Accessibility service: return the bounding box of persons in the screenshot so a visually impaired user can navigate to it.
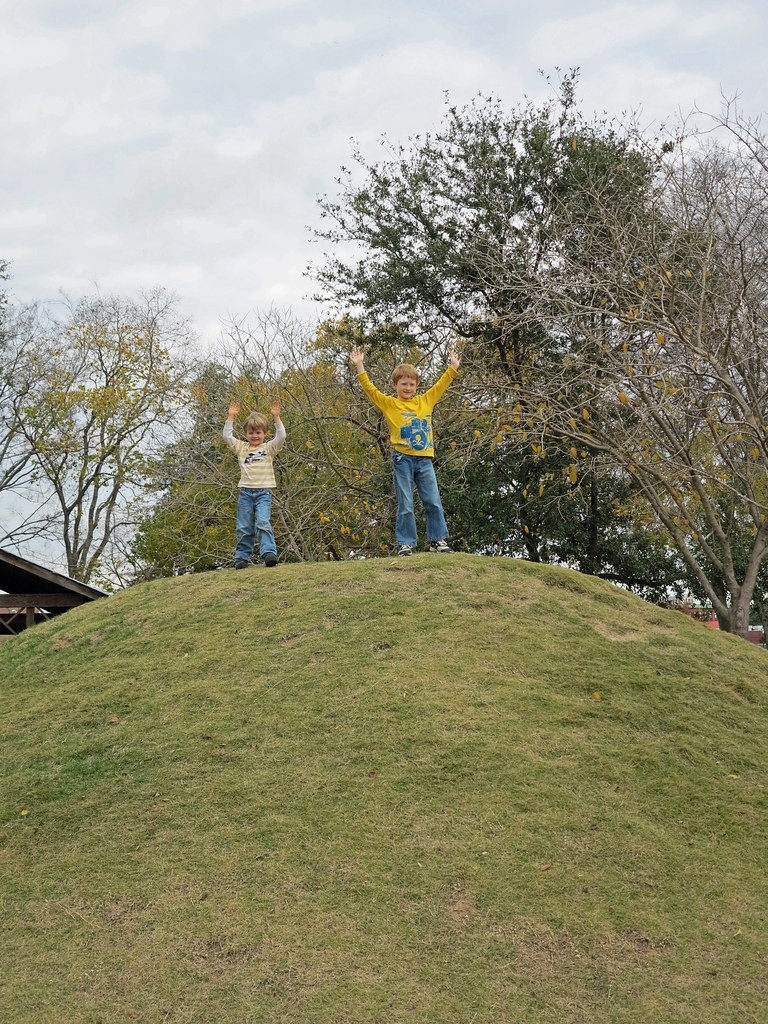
[350,347,461,555]
[223,401,286,570]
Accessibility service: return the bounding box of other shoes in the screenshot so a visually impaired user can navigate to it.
[235,558,248,570]
[265,552,278,567]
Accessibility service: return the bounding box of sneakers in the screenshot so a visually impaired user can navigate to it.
[398,544,413,556]
[429,539,452,552]
